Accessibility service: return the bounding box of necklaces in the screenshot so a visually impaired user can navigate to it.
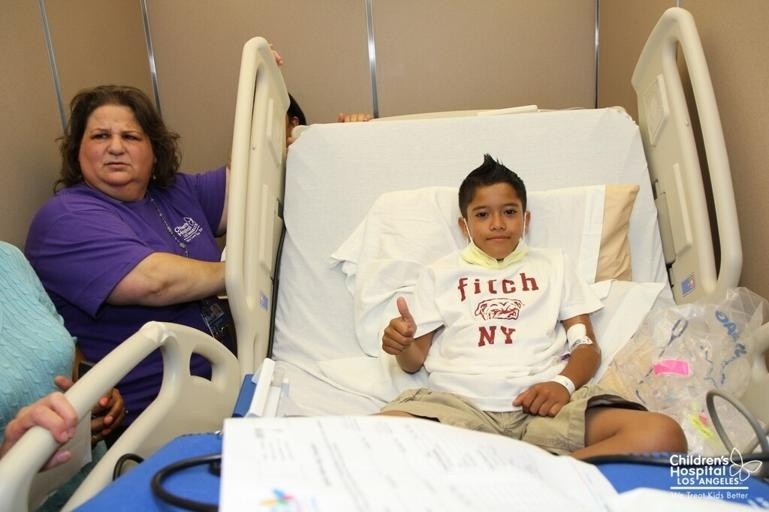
[147,192,190,258]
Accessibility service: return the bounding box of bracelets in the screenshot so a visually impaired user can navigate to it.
[553,375,576,395]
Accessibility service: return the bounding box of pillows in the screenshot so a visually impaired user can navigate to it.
[330,184,644,361]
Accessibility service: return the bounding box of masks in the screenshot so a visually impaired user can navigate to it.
[459,210,530,271]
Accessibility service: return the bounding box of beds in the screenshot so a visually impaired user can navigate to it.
[0,4,769,507]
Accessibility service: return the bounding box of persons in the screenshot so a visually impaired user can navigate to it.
[284,92,375,149]
[382,152,689,458]
[0,239,127,510]
[23,43,283,450]
[0,392,76,471]
